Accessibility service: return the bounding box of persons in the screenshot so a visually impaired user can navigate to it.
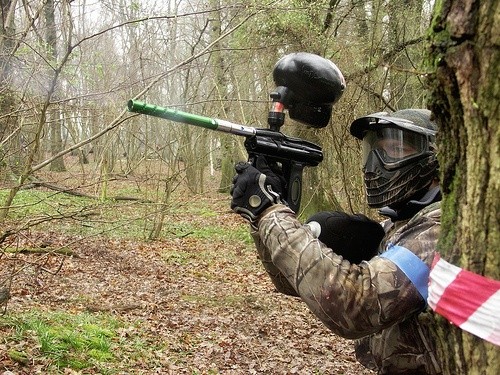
[229,107,445,375]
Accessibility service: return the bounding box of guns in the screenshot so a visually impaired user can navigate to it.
[126,52,346,216]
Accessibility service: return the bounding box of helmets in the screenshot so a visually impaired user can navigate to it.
[350,108,441,209]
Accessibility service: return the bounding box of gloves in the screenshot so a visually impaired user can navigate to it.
[229,154,288,231]
